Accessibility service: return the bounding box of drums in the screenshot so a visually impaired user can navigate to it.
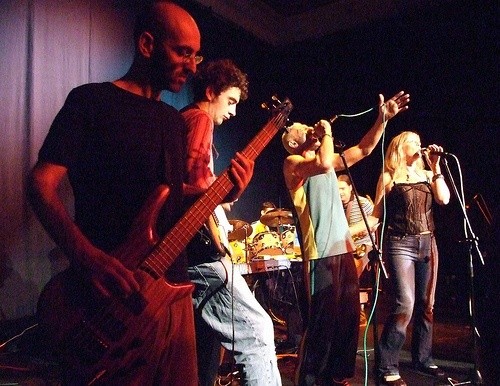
[281,228,300,255]
[249,230,284,258]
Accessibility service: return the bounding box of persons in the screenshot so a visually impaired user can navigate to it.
[372,131,451,386]
[281,90,410,386]
[176,57,283,386]
[24,2,252,386]
[336,175,378,281]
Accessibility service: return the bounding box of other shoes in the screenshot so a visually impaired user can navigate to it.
[329,376,353,386]
[276,342,300,354]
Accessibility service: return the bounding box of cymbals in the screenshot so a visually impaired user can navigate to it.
[226,219,254,242]
[261,211,294,225]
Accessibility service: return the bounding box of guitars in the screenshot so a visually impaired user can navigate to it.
[36,93,294,381]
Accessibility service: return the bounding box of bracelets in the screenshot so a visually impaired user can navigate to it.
[322,134,334,141]
[432,174,444,183]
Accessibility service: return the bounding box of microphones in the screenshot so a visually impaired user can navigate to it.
[466,193,479,208]
[312,115,338,138]
[420,148,453,156]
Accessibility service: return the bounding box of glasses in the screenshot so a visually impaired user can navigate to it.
[154,37,203,65]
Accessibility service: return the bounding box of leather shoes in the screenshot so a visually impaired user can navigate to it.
[381,375,407,386]
[419,365,446,376]
